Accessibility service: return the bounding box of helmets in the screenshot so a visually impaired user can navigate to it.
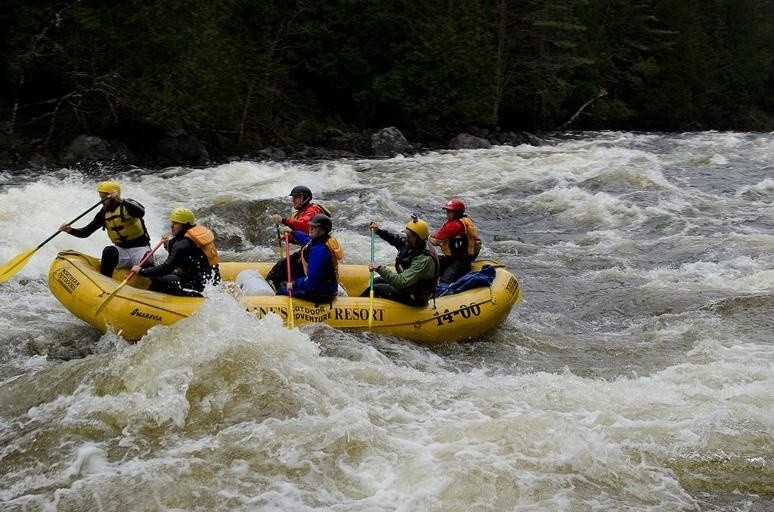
[307,214,332,232]
[97,181,121,194]
[289,186,312,199]
[442,199,465,212]
[169,209,195,225]
[406,219,429,240]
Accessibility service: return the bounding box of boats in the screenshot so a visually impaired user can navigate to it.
[49,250,520,344]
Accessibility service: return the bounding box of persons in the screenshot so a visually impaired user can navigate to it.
[429,199,480,285]
[359,219,439,307]
[276,214,343,304]
[265,185,332,290]
[58,182,155,277]
[130,208,221,297]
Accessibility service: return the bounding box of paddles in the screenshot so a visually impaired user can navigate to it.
[284,232,294,328]
[0,190,118,283]
[94,235,169,315]
[369,221,373,329]
